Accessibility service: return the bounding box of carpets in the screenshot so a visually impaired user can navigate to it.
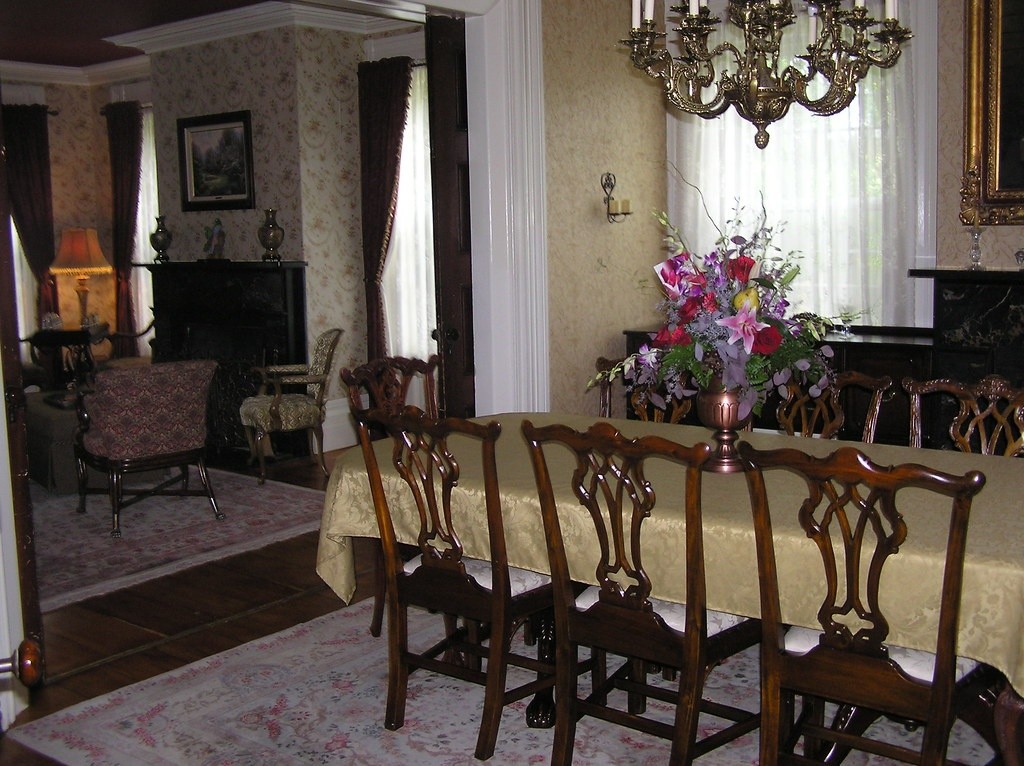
[5,588,997,766]
[29,463,326,612]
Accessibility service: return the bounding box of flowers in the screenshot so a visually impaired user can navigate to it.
[582,185,860,383]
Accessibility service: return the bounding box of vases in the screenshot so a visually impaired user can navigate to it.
[258,208,285,261]
[150,217,170,261]
[966,228,986,265]
[695,354,752,472]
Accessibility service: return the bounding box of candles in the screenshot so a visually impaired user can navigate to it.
[974,210,979,228]
[608,197,630,213]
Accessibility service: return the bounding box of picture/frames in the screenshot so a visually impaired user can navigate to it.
[177,109,255,212]
[959,0,1024,225]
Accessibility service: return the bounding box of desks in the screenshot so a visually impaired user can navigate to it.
[31,323,107,395]
[315,414,1024,691]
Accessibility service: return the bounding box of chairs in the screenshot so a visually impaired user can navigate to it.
[241,327,1024,765]
[69,361,225,536]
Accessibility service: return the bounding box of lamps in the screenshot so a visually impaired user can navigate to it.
[51,227,112,326]
[617,0,914,149]
